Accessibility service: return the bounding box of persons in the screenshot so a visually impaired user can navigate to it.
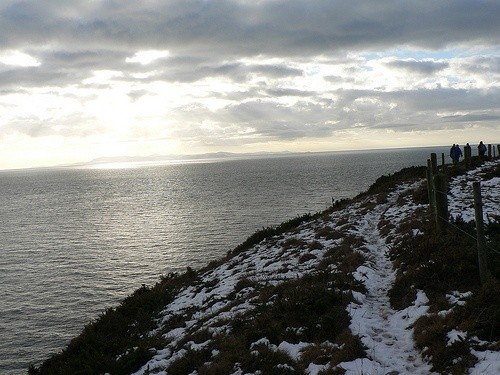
[466,142,471,157]
[478,141,487,157]
[450,144,457,164]
[455,145,463,163]
